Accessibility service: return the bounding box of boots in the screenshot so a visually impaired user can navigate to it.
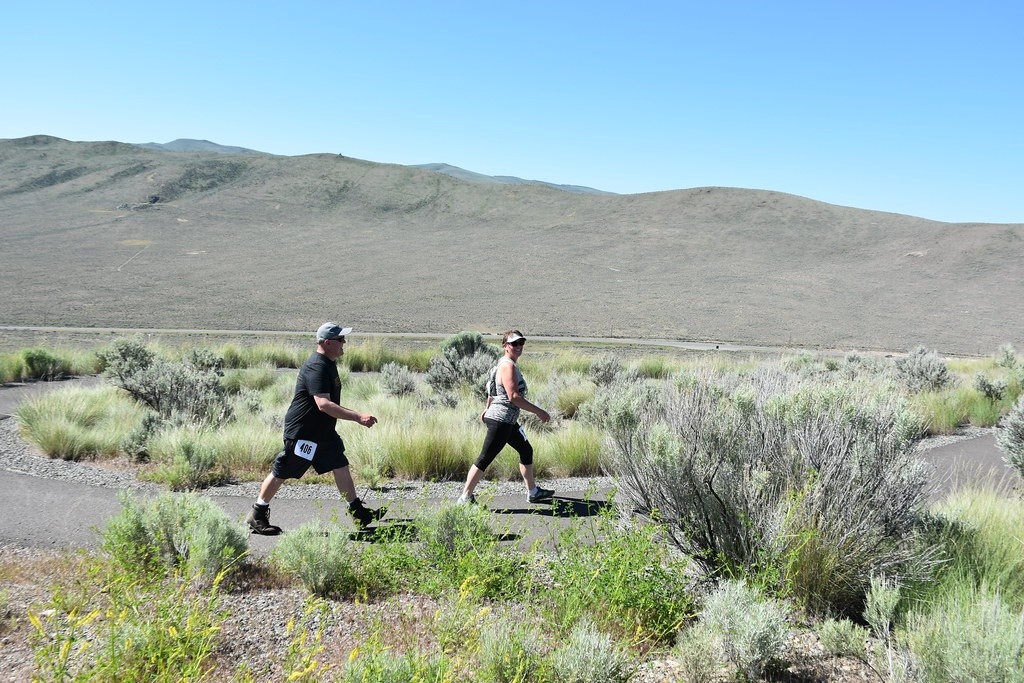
[347,498,389,530]
[242,503,284,535]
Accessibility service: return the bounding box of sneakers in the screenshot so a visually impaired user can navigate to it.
[457,494,487,510]
[526,487,555,503]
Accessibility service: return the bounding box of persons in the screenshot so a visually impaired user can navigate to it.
[246,323,387,535]
[458,330,555,507]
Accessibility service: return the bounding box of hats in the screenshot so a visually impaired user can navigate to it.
[316,322,352,343]
[505,332,526,342]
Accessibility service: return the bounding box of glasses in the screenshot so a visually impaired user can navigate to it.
[323,335,344,344]
[506,340,525,347]
